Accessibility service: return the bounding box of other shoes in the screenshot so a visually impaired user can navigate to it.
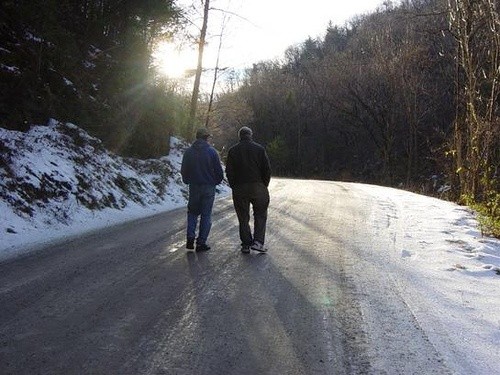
[251,241,268,253]
[195,244,210,253]
[240,245,250,254]
[185,241,194,249]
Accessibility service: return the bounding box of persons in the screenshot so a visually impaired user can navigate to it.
[181,130,224,253]
[225,126,271,254]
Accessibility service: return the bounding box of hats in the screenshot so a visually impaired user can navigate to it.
[238,127,254,138]
[195,127,213,138]
